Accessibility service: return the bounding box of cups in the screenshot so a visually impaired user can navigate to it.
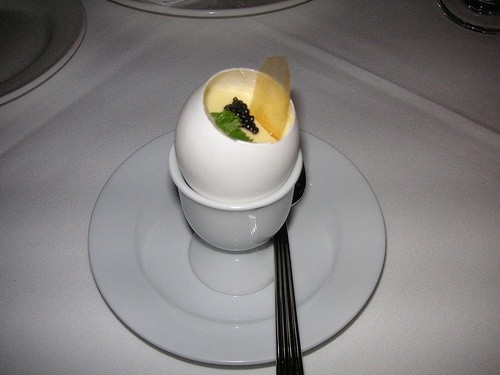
[169,142,303,297]
[436,0,500,37]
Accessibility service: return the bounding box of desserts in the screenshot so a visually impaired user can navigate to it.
[206,81,292,143]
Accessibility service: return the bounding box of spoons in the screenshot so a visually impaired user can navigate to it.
[274,162,306,375]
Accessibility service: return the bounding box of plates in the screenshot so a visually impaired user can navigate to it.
[109,0,311,17]
[0,0,89,103]
[88,129,387,365]
